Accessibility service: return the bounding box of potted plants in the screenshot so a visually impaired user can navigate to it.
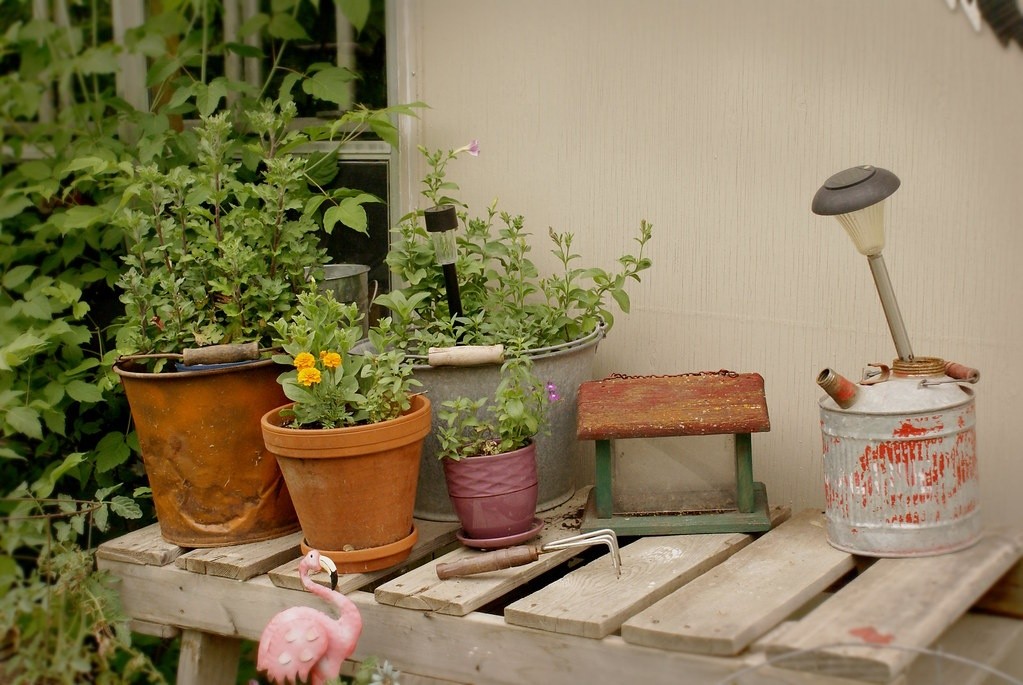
[257,274,435,577]
[110,96,336,551]
[434,335,562,550]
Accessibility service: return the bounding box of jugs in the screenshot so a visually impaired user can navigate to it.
[815,356,986,557]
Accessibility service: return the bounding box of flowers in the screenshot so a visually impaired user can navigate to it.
[284,347,369,431]
[346,136,658,360]
[523,378,562,444]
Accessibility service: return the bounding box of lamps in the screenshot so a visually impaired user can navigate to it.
[422,204,460,266]
[812,164,902,258]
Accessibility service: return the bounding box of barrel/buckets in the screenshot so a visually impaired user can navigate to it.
[301,264,372,339]
